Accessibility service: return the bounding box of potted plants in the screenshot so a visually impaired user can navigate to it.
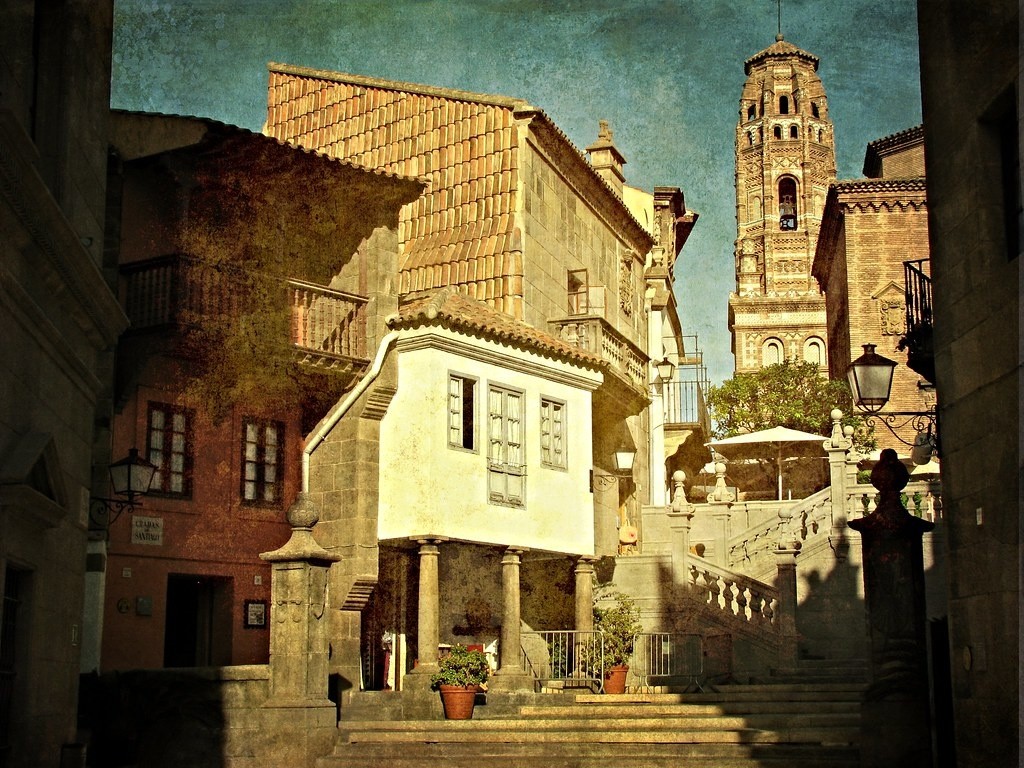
[580,593,645,695]
[429,643,490,720]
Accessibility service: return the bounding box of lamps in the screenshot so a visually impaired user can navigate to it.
[843,342,943,460]
[593,440,637,491]
[88,446,160,528]
[649,357,676,385]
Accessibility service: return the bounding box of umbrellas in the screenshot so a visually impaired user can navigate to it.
[849,447,912,467]
[909,459,940,481]
[703,425,828,501]
